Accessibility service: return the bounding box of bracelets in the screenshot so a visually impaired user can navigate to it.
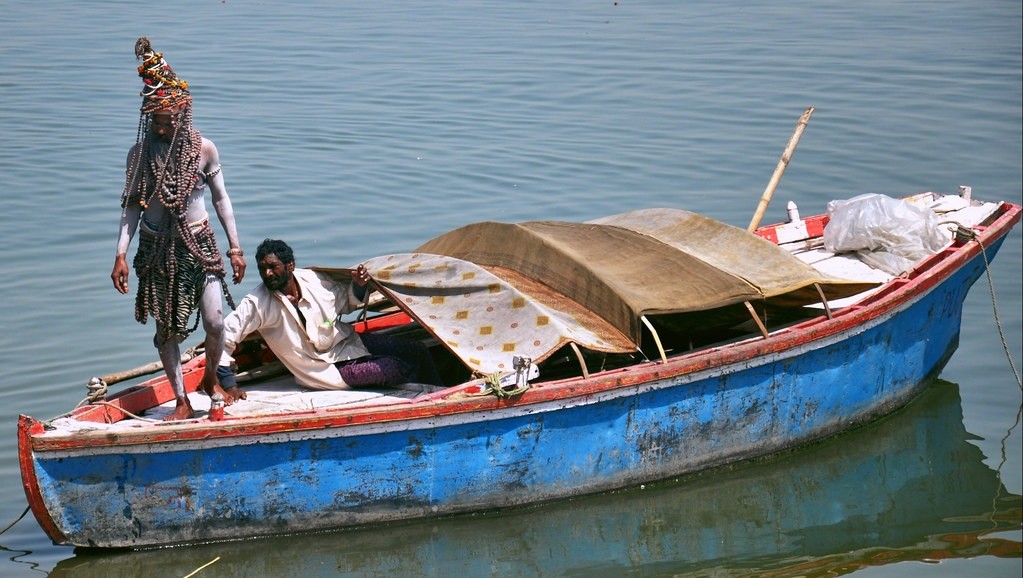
[226,248,244,258]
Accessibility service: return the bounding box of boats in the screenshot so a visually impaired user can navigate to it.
[15,189,1023,550]
[30,376,1021,577]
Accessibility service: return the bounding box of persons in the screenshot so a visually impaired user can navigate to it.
[216,238,446,403]
[111,90,247,422]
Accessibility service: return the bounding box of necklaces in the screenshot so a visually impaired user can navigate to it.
[141,129,203,220]
